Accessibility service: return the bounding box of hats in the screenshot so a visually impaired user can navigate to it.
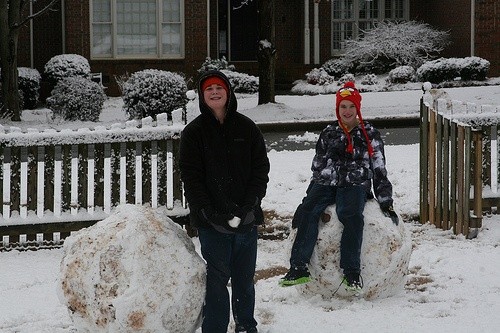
[334,81,361,117]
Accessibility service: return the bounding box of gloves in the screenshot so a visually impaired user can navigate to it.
[381,200,399,225]
[291,202,305,229]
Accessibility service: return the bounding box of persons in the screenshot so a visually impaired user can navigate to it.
[282,81,399,291]
[178,70,270,333]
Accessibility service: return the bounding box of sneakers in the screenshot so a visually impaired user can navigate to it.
[278,266,311,285]
[342,273,361,295]
